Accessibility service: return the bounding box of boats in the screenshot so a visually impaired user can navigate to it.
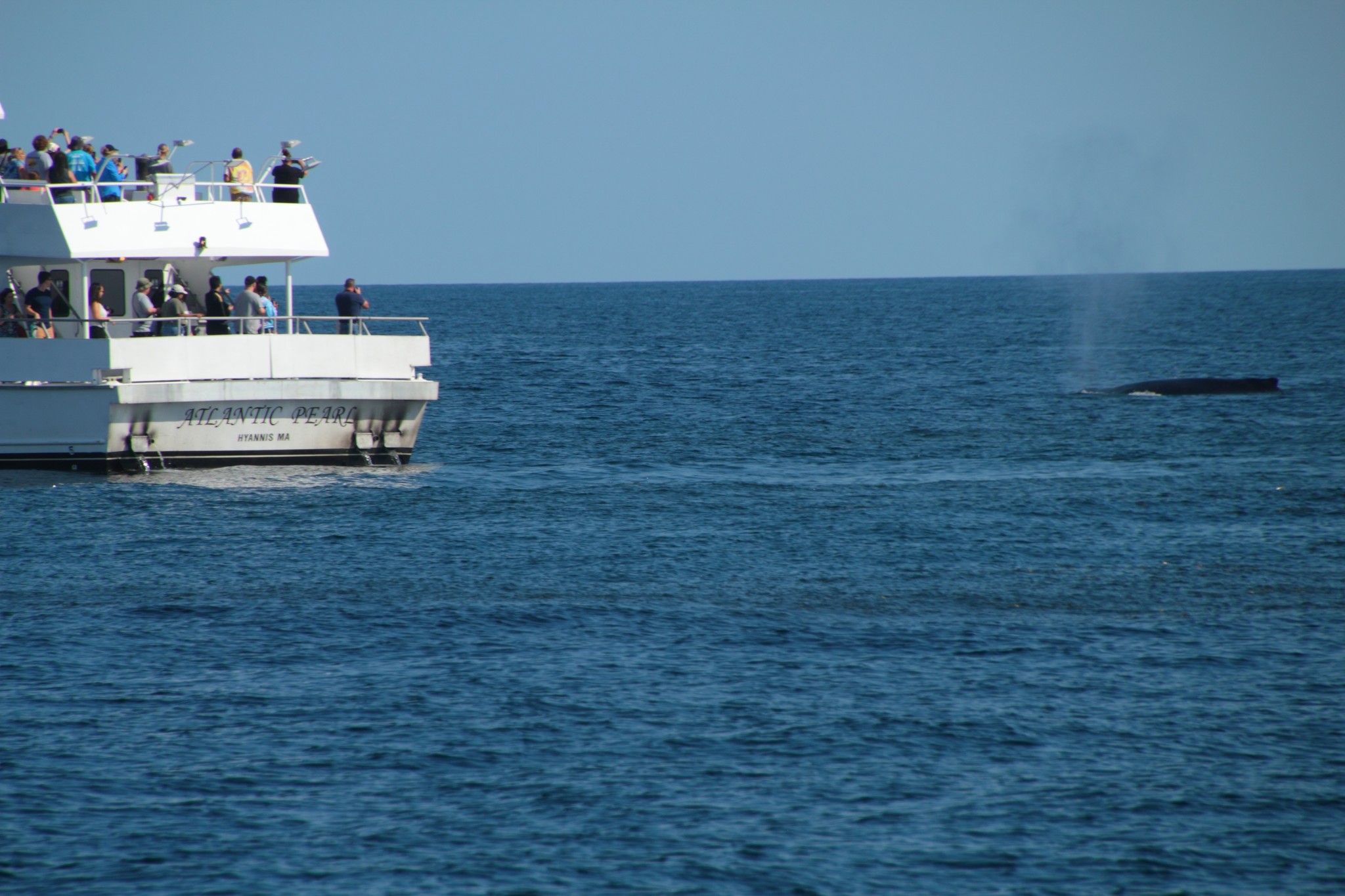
[0,136,444,479]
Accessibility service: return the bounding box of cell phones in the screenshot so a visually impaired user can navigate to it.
[57,128,63,134]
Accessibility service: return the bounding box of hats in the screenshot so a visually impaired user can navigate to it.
[67,135,86,150]
[171,284,189,294]
[245,276,258,285]
[47,142,60,153]
[135,277,154,290]
[102,144,119,154]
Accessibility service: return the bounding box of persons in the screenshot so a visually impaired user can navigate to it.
[223,147,254,203]
[271,152,309,203]
[0,269,279,338]
[335,278,369,335]
[0,127,174,204]
